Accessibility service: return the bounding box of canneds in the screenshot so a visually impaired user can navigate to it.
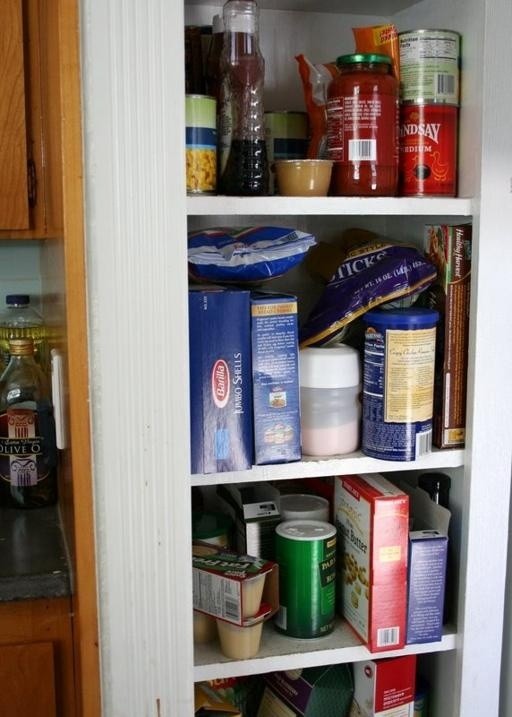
[361,305,440,462]
[187,94,218,193]
[274,493,337,640]
[329,54,400,197]
[263,110,311,196]
[398,27,462,104]
[401,103,459,198]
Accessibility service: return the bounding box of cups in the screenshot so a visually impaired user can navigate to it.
[273,158,337,196]
[195,572,269,659]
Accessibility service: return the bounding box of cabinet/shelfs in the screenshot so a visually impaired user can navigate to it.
[109,2,512,717]
[0,0,86,241]
[6,600,95,717]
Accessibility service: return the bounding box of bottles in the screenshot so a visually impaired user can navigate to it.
[417,470,455,624]
[0,292,62,507]
[206,0,270,194]
[299,342,364,456]
